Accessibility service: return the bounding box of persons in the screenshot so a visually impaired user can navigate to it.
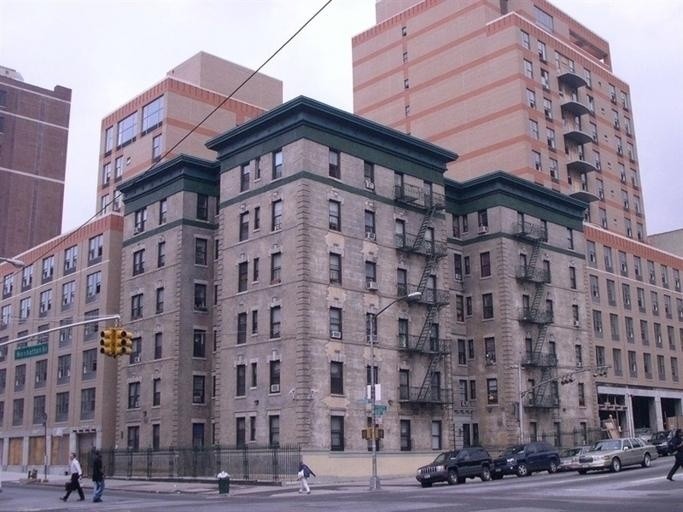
[667,429,683,481]
[59,452,84,502]
[92,449,105,502]
[299,461,316,494]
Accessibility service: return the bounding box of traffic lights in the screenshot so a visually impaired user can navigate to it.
[115,330,132,354]
[561,376,576,385]
[100,328,115,358]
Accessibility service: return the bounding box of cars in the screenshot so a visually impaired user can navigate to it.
[415,430,683,488]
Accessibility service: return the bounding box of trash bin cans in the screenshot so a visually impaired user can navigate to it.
[217,472,230,493]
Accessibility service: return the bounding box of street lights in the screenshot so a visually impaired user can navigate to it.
[370,291,423,491]
